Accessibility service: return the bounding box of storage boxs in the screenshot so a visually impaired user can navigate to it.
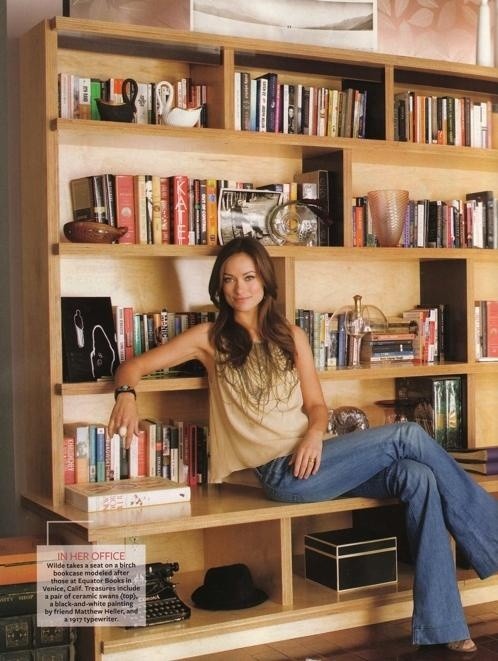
[304,528,400,593]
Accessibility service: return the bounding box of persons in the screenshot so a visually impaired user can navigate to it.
[103,236,497,654]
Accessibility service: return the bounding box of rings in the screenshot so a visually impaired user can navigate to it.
[118,427,127,437]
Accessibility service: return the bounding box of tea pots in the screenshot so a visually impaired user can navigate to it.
[62,219,130,242]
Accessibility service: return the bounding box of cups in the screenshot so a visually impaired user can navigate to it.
[368,187,411,247]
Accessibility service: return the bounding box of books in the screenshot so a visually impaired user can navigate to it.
[455,462,498,476]
[439,377,465,450]
[61,416,211,512]
[395,378,444,450]
[58,67,496,385]
[446,446,497,464]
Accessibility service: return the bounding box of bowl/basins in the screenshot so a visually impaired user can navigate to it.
[94,79,141,123]
[156,82,205,127]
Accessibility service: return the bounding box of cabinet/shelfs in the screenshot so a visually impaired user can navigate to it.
[21,471,498,661]
[20,16,497,507]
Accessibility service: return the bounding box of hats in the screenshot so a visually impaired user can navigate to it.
[191,562,269,610]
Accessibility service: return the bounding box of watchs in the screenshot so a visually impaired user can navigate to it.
[114,384,136,403]
